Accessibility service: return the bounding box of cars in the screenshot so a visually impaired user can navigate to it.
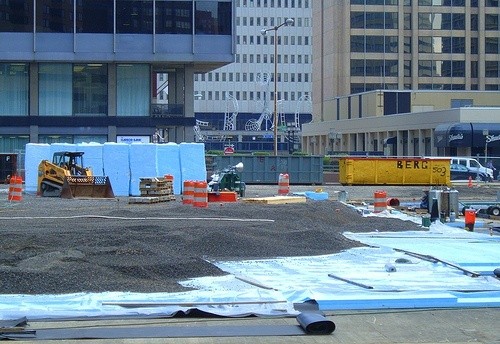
[449,164,491,182]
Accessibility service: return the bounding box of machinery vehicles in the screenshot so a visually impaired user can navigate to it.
[36,151,115,199]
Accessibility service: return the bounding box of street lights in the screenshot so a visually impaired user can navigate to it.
[259,19,297,157]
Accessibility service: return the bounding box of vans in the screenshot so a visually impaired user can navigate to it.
[422,156,494,182]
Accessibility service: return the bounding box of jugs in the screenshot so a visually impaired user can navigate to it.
[450,209,455,222]
[441,210,446,222]
